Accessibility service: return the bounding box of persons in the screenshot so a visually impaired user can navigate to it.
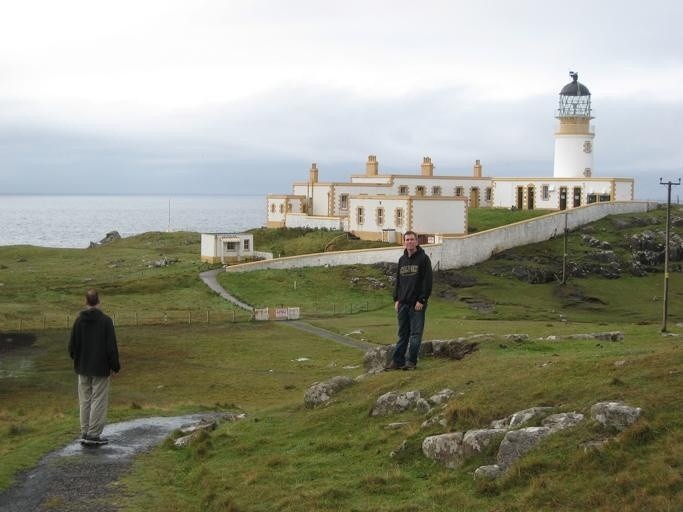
[66,288,121,448]
[384,230,433,371]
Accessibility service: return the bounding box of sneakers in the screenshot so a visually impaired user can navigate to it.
[403,366,417,370]
[386,361,402,369]
[81,432,108,445]
